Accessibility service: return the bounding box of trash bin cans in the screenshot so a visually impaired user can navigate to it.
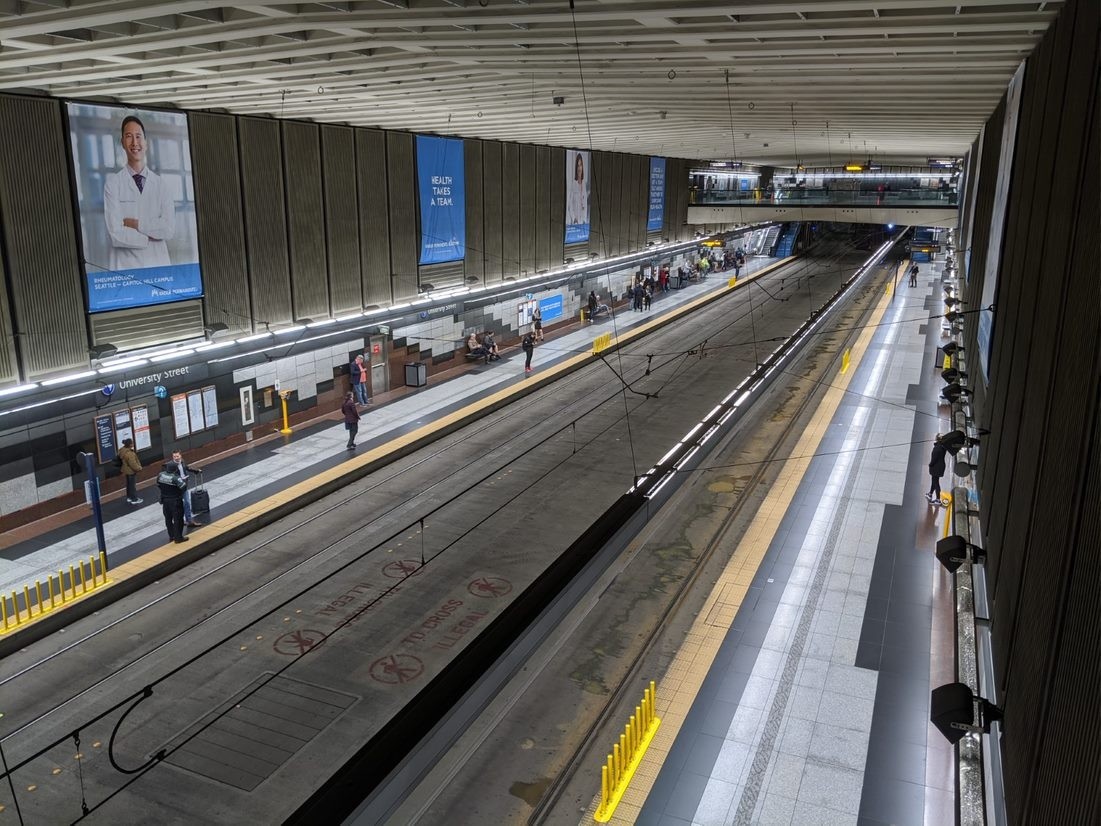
[403,362,427,388]
[670,276,680,289]
[941,270,950,281]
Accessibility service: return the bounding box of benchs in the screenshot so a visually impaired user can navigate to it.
[465,342,498,364]
[584,296,630,319]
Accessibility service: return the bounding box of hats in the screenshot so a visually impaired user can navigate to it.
[167,460,178,472]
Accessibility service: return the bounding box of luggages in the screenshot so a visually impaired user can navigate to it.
[191,471,211,514]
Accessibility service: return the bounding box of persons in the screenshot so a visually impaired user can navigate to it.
[762,182,784,199]
[587,290,611,323]
[156,461,189,544]
[878,183,890,200]
[706,176,712,197]
[523,332,537,372]
[627,272,656,312]
[569,153,588,225]
[924,433,947,506]
[118,439,144,505]
[342,392,361,450]
[468,329,502,365]
[660,245,747,292]
[350,355,372,407]
[532,308,544,341]
[172,449,203,528]
[908,262,919,288]
[103,115,176,271]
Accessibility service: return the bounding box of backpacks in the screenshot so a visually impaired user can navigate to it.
[111,448,131,472]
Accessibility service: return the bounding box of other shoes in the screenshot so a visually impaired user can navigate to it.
[169,534,189,544]
[490,352,502,361]
[925,493,932,500]
[360,400,370,406]
[587,319,594,322]
[184,519,203,526]
[929,499,942,505]
[525,367,533,372]
[485,361,491,364]
[126,496,143,504]
[347,443,356,449]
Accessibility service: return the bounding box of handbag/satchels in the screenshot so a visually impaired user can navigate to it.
[345,420,349,430]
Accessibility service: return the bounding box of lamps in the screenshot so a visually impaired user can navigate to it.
[842,164,866,174]
[567,257,575,264]
[941,368,968,384]
[88,342,118,361]
[648,241,655,247]
[796,163,804,170]
[421,283,436,293]
[205,321,230,339]
[945,238,955,273]
[865,160,884,172]
[591,252,598,258]
[935,535,989,572]
[944,296,962,307]
[945,311,965,322]
[942,340,966,356]
[942,429,980,456]
[930,680,1000,741]
[466,276,480,284]
[944,286,958,294]
[943,382,973,405]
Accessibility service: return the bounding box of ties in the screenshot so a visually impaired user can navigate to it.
[133,175,144,191]
